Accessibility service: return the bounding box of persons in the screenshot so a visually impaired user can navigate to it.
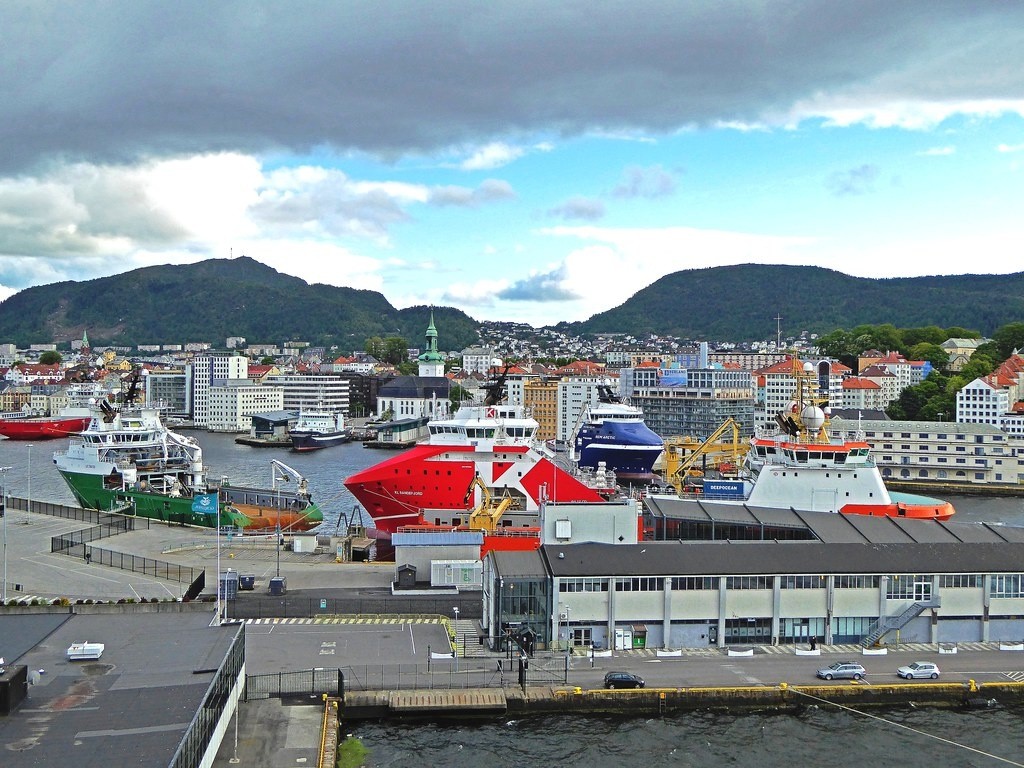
[86,552,91,564]
[810,636,816,650]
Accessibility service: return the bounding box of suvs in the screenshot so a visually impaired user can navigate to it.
[897,661,940,680]
[816,661,866,680]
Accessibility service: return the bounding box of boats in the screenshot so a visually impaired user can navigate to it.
[288,386,353,450]
[343,414,644,562]
[568,369,666,486]
[418,367,557,460]
[650,347,955,536]
[52,368,323,533]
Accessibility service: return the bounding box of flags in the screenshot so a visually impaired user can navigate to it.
[191,493,218,513]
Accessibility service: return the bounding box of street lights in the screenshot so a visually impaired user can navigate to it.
[453,607,459,672]
[1,467,13,599]
[26,445,34,520]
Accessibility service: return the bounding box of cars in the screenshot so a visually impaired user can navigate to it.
[603,671,645,689]
